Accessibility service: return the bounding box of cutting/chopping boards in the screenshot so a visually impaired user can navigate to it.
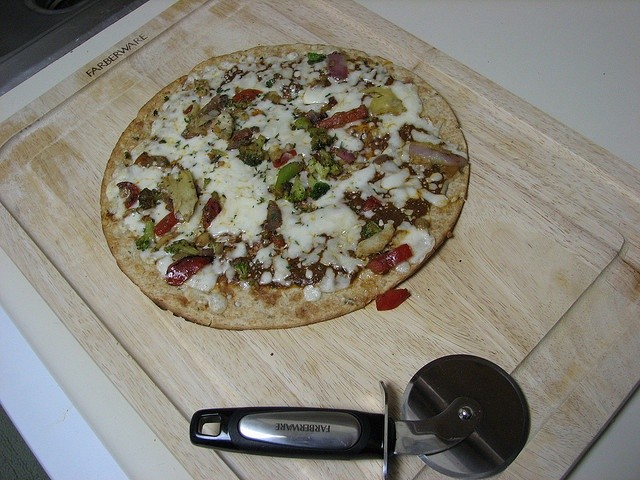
[0,0,640,480]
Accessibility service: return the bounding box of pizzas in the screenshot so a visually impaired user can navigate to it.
[99,43,471,329]
